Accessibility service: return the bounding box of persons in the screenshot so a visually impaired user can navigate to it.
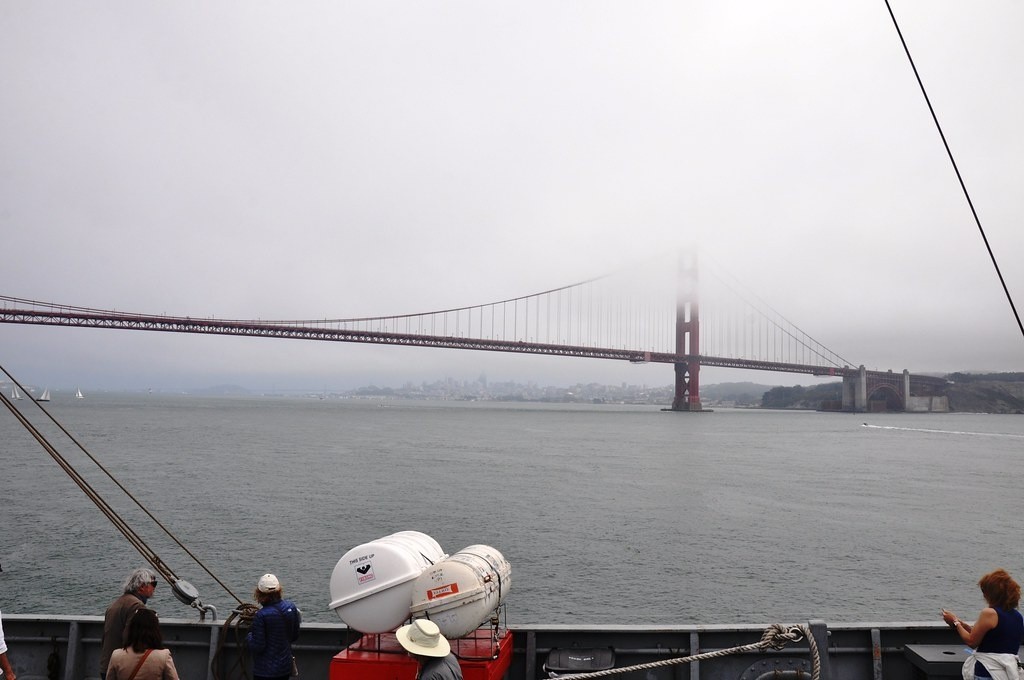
[101,569,156,680]
[243,571,300,680]
[0,564,17,680]
[941,569,1024,680]
[106,610,180,680]
[395,619,463,680]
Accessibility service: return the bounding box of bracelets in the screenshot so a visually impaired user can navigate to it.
[952,622,960,629]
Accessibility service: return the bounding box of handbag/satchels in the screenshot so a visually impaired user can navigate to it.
[289,656,299,677]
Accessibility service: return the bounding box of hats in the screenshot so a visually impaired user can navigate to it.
[395,619,451,658]
[257,573,280,593]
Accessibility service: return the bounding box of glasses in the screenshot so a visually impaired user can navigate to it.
[135,609,157,617]
[147,580,157,588]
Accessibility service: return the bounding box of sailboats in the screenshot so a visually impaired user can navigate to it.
[36,386,51,402]
[75,387,84,398]
[11,385,23,400]
